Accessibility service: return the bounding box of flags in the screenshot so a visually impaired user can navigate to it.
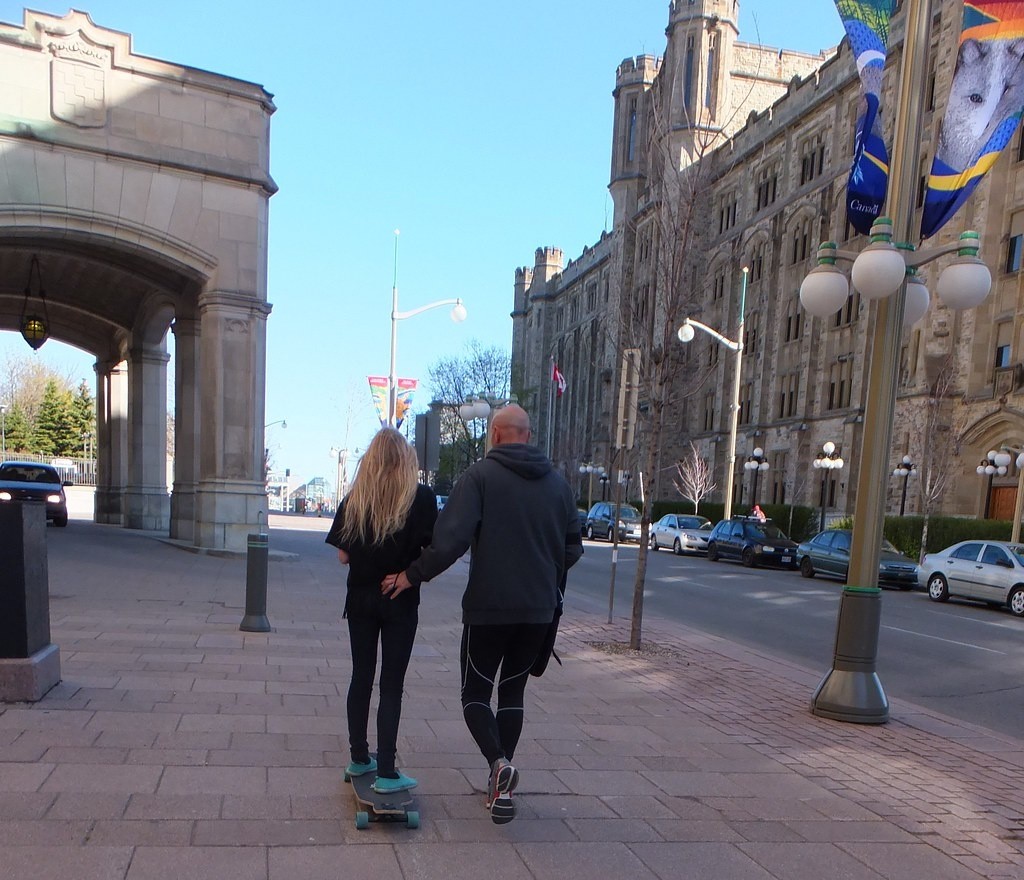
[550,364,567,398]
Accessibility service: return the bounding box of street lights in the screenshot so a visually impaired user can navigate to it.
[812,441,844,531]
[744,447,769,512]
[677,266,750,520]
[800,215,993,726]
[893,454,918,517]
[975,450,1012,520]
[0,404,7,459]
[387,227,466,427]
[578,461,604,512]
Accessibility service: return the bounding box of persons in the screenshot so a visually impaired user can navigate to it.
[754,504,766,523]
[379,402,587,827]
[317,503,321,517]
[325,425,437,798]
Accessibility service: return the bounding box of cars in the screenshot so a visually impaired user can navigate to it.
[577,501,652,544]
[917,539,1024,617]
[650,514,715,556]
[795,528,917,591]
[0,462,73,527]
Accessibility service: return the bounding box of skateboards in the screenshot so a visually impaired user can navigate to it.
[343,751,419,830]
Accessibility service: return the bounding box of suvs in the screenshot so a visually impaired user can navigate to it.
[705,514,799,571]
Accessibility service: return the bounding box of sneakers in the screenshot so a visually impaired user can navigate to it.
[374,767,417,792]
[488,757,519,824]
[346,757,377,776]
